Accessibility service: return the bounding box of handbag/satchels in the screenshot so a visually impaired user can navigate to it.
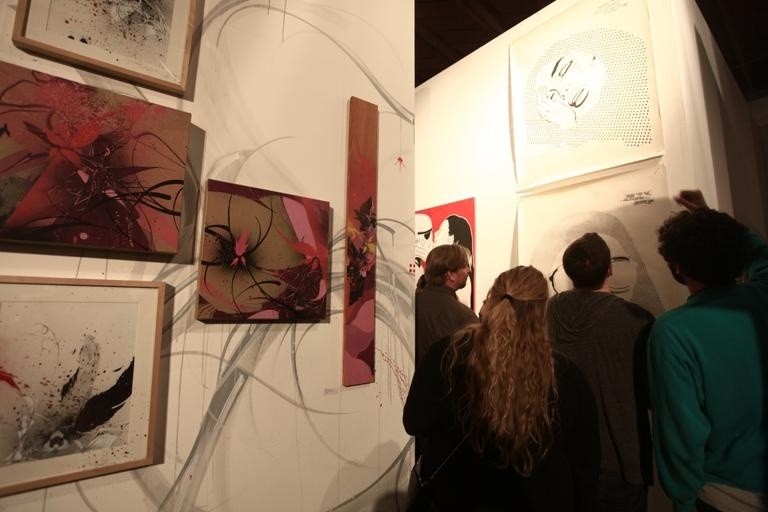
[404,453,430,512]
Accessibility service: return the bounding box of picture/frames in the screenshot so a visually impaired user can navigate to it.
[12,0,197,94]
[0,275,166,496]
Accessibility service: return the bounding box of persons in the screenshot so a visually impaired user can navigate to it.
[396,265,603,508]
[398,243,481,468]
[544,229,660,510]
[535,55,599,129]
[415,214,474,309]
[638,188,767,512]
[415,212,434,291]
